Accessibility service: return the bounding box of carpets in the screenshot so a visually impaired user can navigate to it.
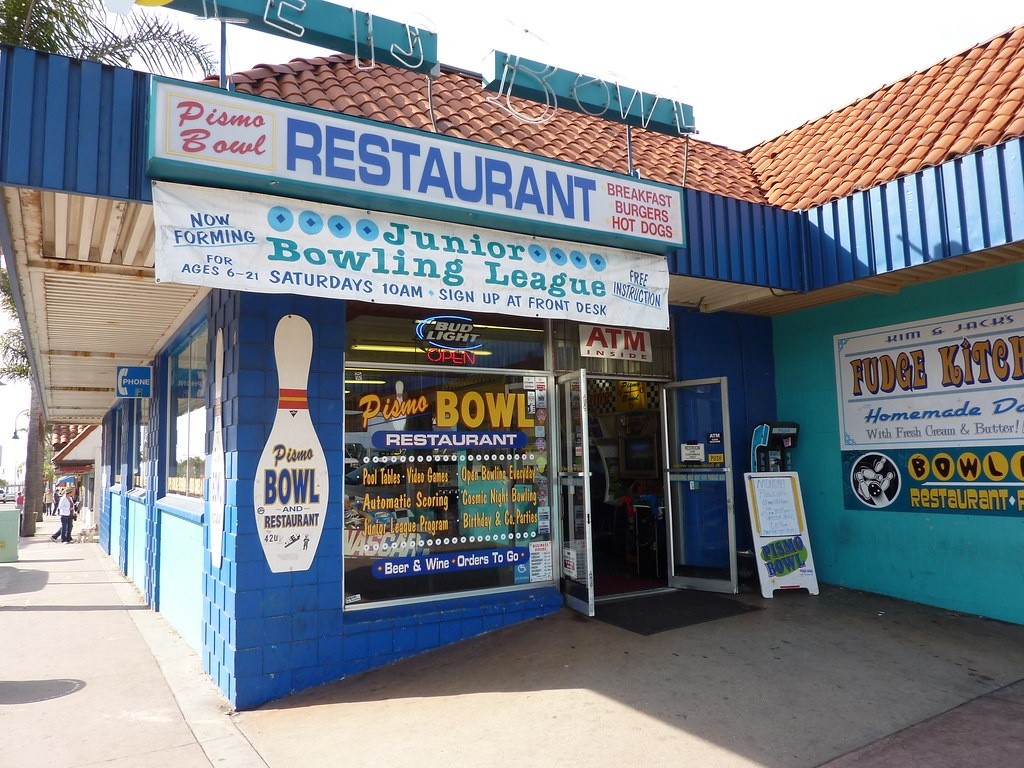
[589,588,768,636]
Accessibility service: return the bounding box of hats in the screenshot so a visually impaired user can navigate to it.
[66,488,74,494]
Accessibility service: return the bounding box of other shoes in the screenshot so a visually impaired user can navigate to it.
[52,514,56,516]
[69,540,75,543]
[50,536,57,542]
[61,541,67,544]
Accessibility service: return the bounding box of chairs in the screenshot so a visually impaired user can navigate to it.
[633,505,666,578]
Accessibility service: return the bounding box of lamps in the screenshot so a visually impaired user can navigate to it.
[12,409,30,439]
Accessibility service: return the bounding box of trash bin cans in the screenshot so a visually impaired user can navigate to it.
[0,508,21,562]
[20,512,39,536]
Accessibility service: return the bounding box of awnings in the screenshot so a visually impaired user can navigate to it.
[58,476,75,482]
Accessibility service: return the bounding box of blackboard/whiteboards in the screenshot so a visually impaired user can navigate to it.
[749,474,804,537]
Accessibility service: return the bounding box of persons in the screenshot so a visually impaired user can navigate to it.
[16,491,24,512]
[44,487,79,544]
